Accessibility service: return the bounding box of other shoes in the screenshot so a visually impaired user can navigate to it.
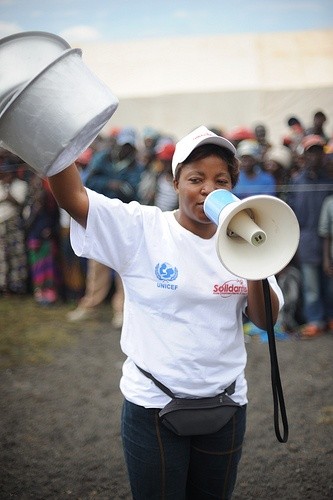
[112,312,123,330]
[244,321,262,336]
[67,307,97,321]
[262,325,287,342]
[301,324,324,337]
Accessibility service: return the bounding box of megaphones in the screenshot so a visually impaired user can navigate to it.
[203,188,301,280]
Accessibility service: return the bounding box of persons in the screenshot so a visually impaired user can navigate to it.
[42,127,285,500]
[0,111,333,342]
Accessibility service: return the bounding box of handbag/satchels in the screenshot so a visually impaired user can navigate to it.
[134,364,241,435]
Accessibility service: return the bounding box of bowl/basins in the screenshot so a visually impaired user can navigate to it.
[0,48,119,178]
[0,30,71,112]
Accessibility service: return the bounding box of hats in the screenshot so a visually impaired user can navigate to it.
[116,130,135,149]
[172,126,236,177]
[303,134,324,152]
[265,144,291,169]
[236,139,261,161]
